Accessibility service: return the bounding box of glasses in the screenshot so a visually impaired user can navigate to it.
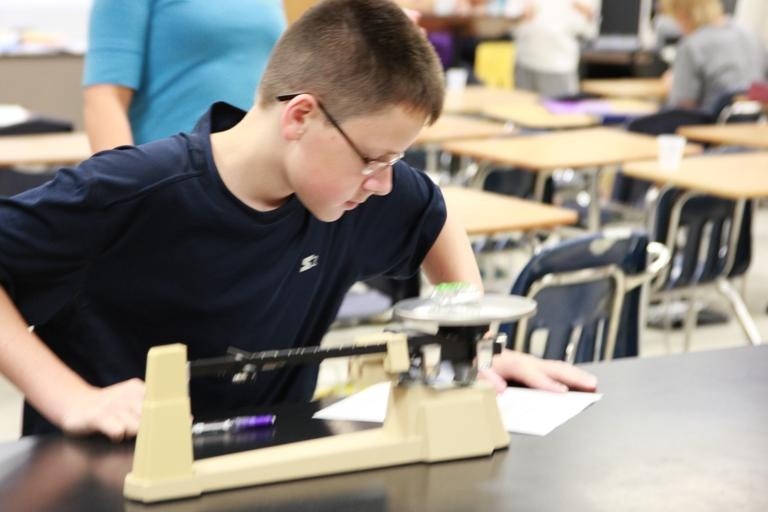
[276,90,407,178]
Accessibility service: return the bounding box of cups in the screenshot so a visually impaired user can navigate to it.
[657,134,687,174]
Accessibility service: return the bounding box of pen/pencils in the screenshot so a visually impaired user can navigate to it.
[192,414,276,432]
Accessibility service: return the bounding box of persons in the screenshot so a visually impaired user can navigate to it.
[80,0,289,158]
[0,0,597,438]
[648,9,681,48]
[657,0,752,112]
[502,0,600,97]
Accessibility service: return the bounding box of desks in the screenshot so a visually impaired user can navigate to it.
[2,73,768,510]
